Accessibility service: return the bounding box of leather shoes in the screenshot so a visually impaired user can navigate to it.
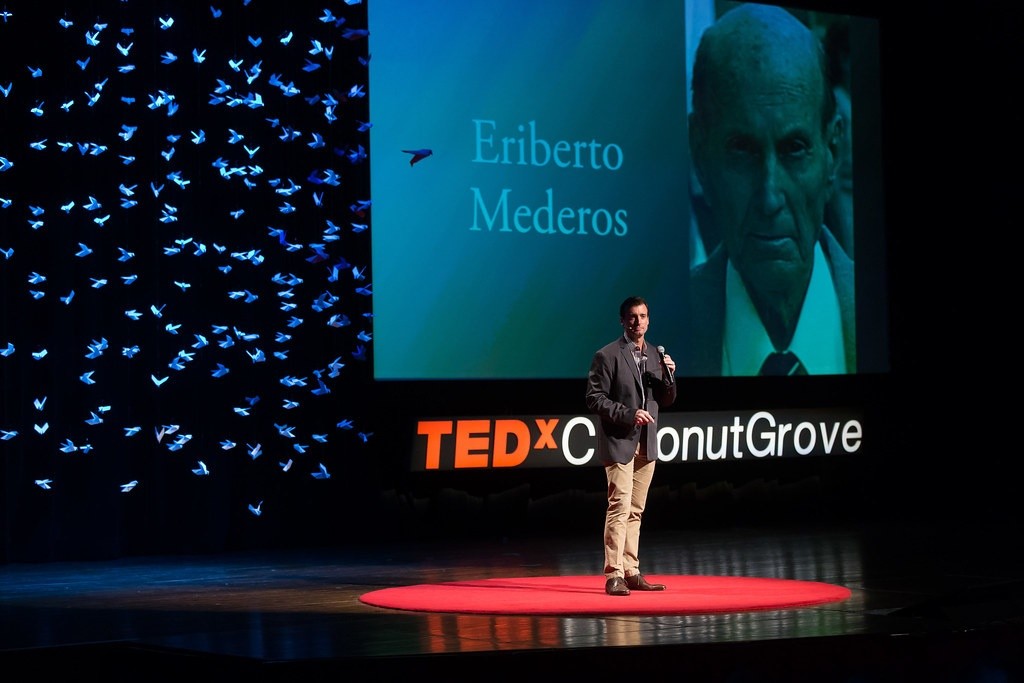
[624,573,665,590]
[605,576,631,596]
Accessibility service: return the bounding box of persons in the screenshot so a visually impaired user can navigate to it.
[586,296,678,595]
[645,3,859,376]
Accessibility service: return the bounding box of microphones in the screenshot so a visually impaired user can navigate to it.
[656,346,674,384]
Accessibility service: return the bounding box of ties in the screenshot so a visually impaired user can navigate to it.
[760,351,809,376]
